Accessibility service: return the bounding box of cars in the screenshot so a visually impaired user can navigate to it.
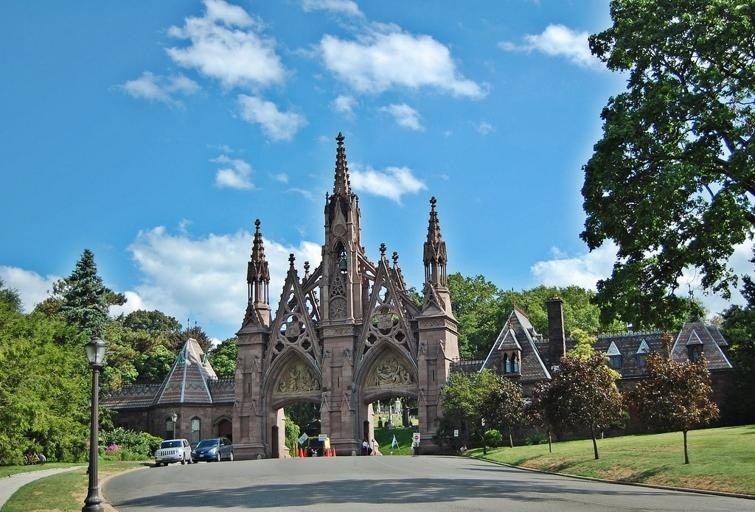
[191,436,233,461]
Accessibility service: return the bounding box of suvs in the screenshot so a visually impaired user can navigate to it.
[154,438,192,466]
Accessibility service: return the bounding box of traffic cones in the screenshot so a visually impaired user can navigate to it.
[327,448,330,457]
[300,448,303,457]
[332,448,336,456]
[303,448,308,457]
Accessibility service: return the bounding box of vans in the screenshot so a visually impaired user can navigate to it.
[307,437,330,456]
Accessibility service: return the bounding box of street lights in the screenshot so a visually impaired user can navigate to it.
[81,331,108,512]
[171,412,178,439]
